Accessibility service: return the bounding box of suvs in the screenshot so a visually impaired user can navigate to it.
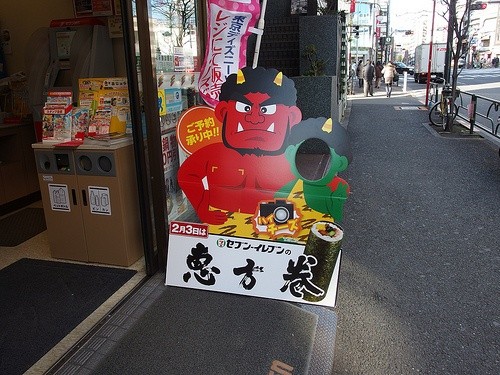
[393,62,414,76]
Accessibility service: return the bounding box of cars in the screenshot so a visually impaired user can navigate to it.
[451,59,464,68]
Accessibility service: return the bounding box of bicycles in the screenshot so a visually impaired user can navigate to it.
[428,77,460,131]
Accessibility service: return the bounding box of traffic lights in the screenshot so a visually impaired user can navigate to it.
[379,37,385,45]
[471,4,487,10]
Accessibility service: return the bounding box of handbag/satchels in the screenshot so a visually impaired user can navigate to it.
[393,75,399,82]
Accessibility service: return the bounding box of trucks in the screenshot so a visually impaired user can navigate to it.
[414,43,447,83]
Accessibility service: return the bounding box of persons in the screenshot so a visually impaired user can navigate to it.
[374,61,384,88]
[356,60,364,88]
[380,61,399,98]
[352,62,357,80]
[362,59,375,97]
